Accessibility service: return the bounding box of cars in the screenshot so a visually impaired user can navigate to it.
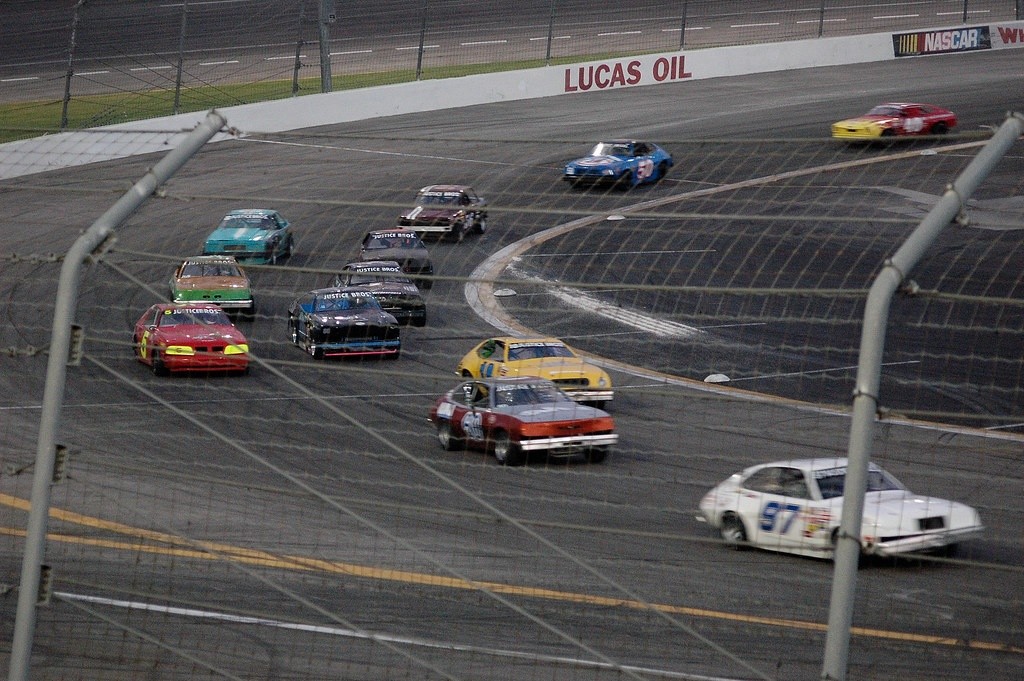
[699,457,984,570]
[427,376,619,464]
[203,209,294,264]
[133,301,251,376]
[400,183,488,242]
[168,256,255,318]
[457,336,615,408]
[831,102,958,150]
[288,289,402,362]
[562,140,674,190]
[329,260,427,327]
[365,228,433,287]
